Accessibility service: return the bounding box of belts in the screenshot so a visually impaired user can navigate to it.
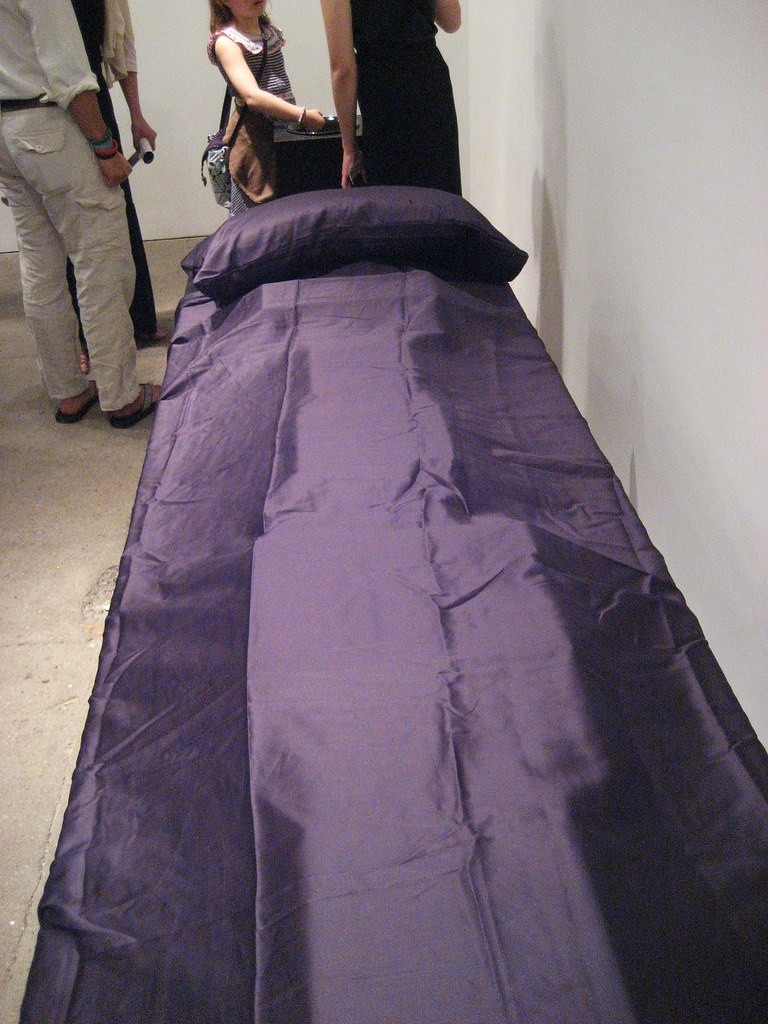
[0,96,57,112]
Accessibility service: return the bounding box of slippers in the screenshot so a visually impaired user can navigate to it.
[110,384,156,428]
[55,380,98,424]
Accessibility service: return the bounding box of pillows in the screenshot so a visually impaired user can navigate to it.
[178,184,528,295]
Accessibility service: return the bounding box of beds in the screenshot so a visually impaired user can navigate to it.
[18,248,767,1024]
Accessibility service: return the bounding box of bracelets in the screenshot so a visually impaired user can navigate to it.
[298,107,306,125]
[90,129,118,160]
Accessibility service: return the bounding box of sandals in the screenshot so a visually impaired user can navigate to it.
[139,331,167,342]
[81,349,89,375]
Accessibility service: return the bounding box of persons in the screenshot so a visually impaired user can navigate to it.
[320,0,462,198]
[66,0,168,372]
[206,0,324,219]
[0,0,162,430]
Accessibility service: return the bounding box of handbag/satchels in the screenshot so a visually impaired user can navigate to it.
[200,131,232,207]
